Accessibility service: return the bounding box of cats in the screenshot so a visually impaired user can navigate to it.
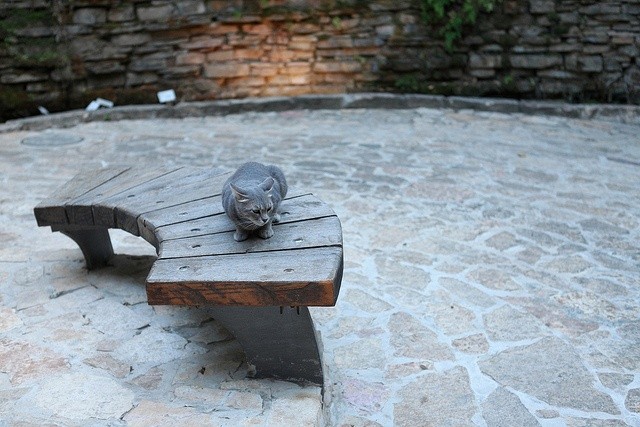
[221,161,288,242]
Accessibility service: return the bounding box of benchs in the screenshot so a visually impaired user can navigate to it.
[34,165,343,308]
